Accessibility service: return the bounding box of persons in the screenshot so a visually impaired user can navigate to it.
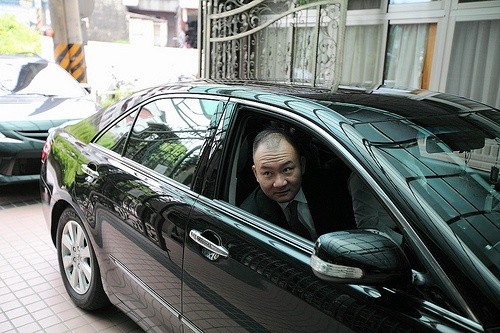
[349,155,430,245]
[238,127,348,243]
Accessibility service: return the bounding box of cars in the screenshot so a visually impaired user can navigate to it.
[0,52,103,188]
[40,79,500,333]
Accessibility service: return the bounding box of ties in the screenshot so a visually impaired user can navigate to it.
[287,200,313,243]
